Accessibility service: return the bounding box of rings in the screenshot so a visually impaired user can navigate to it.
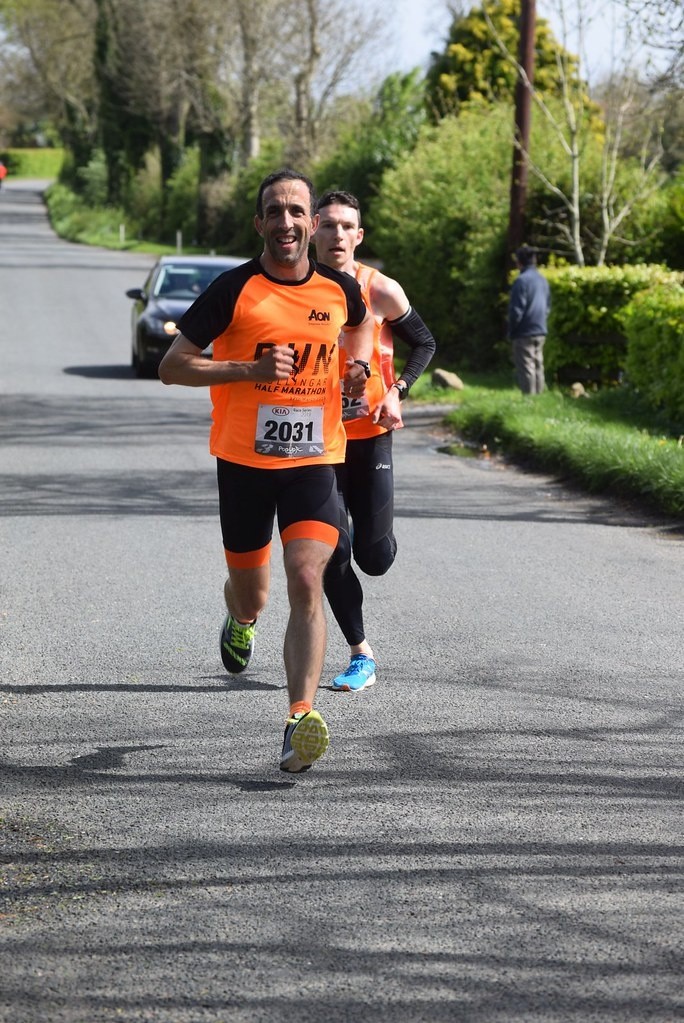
[350,387,353,393]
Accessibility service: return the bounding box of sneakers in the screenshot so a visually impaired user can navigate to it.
[279,710,329,773]
[332,654,376,691]
[220,614,257,673]
[348,521,355,546]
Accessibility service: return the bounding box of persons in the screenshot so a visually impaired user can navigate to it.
[508,248,552,394]
[158,170,374,774]
[0,162,7,187]
[310,191,437,693]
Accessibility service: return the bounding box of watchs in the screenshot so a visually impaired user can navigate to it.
[394,384,408,399]
[355,360,371,378]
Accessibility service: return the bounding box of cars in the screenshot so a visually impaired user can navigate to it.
[125,256,248,380]
